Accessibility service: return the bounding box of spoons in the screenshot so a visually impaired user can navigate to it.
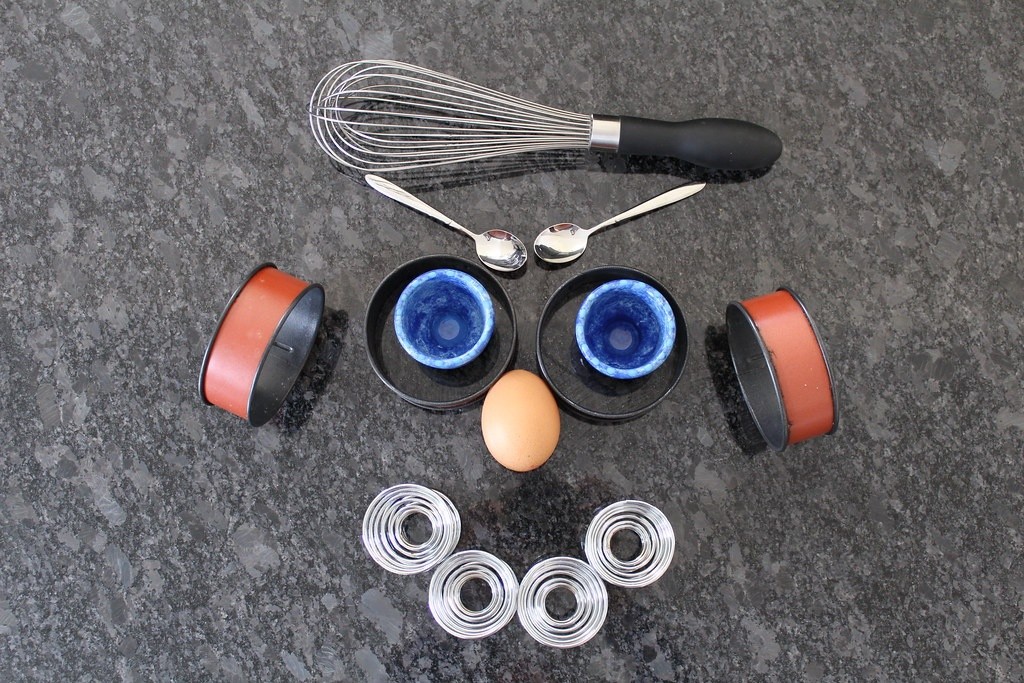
[533,179,707,263]
[365,173,528,272]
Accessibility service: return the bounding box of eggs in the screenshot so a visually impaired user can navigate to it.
[481,369,560,472]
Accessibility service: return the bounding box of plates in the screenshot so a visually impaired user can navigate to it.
[364,254,517,409]
[537,265,688,420]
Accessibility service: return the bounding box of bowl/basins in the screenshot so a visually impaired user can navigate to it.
[393,269,495,369]
[576,278,676,378]
